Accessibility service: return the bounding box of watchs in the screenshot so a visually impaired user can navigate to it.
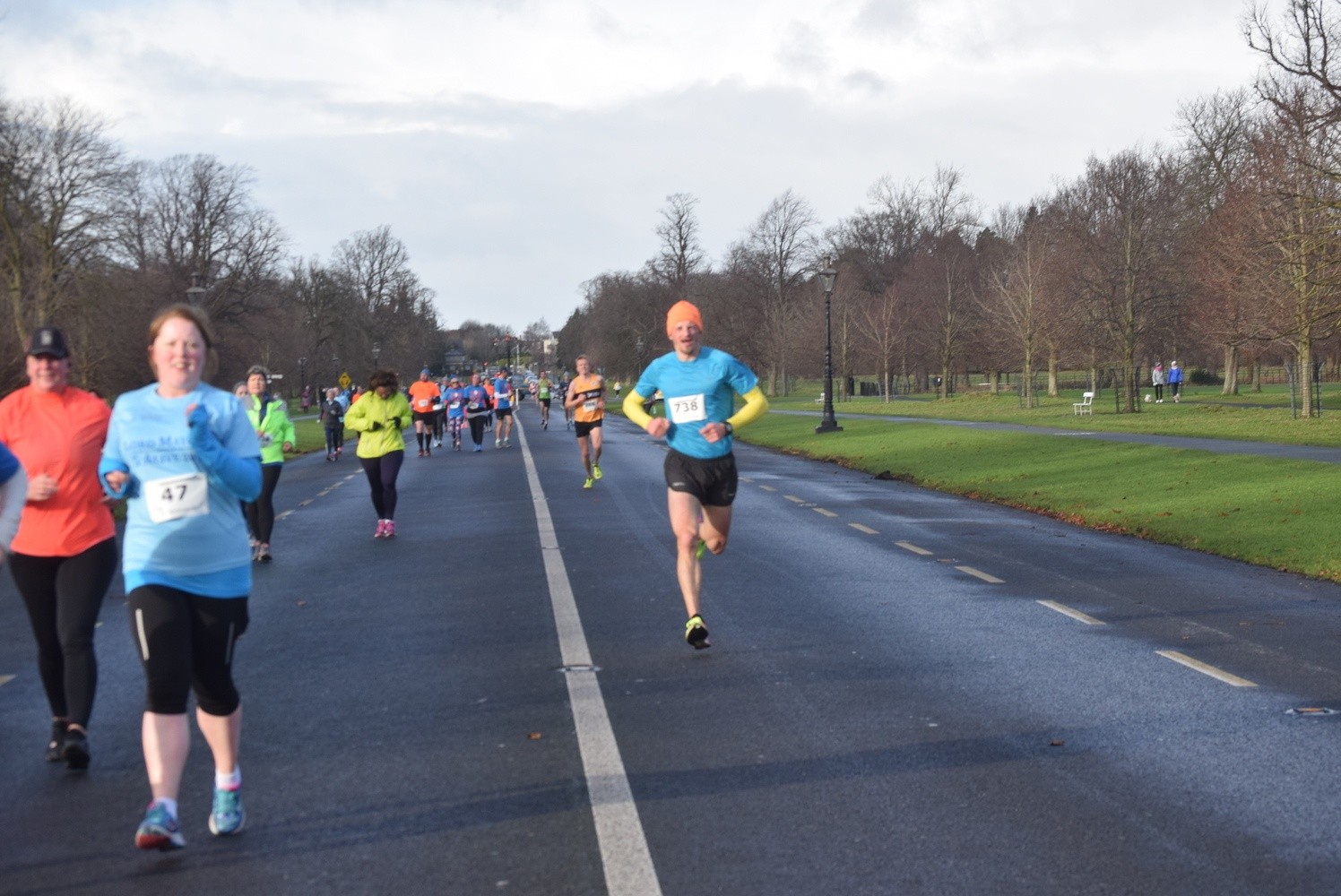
[721,421,733,436]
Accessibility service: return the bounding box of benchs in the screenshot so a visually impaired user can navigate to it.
[1072,392,1095,414]
[814,392,825,404]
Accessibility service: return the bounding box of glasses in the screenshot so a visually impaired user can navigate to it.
[452,381,459,384]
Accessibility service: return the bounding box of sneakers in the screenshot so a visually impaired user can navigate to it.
[374,521,386,537]
[382,522,396,538]
[592,460,603,479]
[209,779,247,835]
[695,539,706,559]
[685,616,711,650]
[135,803,186,852]
[417,419,575,457]
[252,545,259,562]
[583,479,593,488]
[258,547,270,562]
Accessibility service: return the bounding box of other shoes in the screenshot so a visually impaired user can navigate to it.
[63,729,90,776]
[45,722,68,761]
[333,452,338,461]
[326,456,331,461]
[337,449,342,454]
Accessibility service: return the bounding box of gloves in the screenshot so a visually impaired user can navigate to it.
[387,416,401,429]
[373,421,384,431]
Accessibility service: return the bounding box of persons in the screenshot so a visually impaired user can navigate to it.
[1167,360,1183,403]
[565,354,609,488]
[613,382,621,398]
[622,300,769,649]
[559,372,574,428]
[1152,362,1165,403]
[96,302,262,852]
[0,327,117,767]
[234,364,555,562]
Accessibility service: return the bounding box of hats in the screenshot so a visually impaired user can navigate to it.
[421,369,430,375]
[451,378,458,383]
[499,366,507,374]
[23,326,71,358]
[667,301,703,336]
